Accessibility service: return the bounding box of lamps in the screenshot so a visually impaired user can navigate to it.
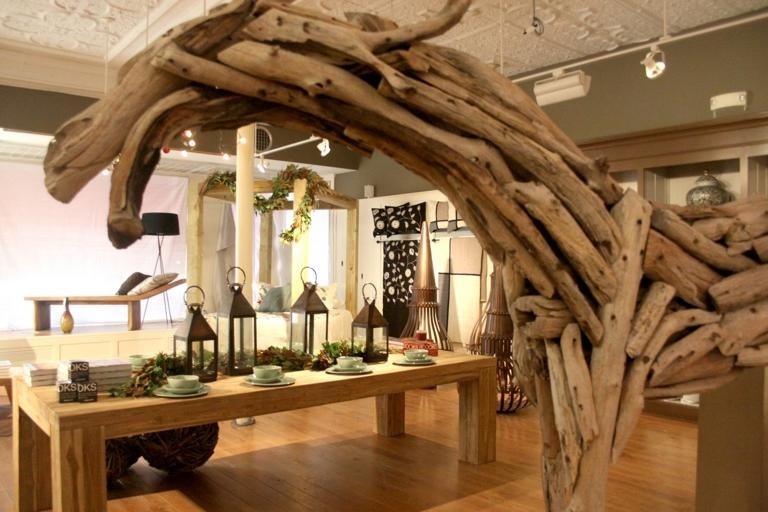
[256,136,330,174]
[639,45,665,80]
[140,211,180,326]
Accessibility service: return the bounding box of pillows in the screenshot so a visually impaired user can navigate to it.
[114,272,151,295]
[127,272,178,295]
[255,283,278,308]
[258,285,291,312]
[386,202,426,238]
[316,284,337,311]
[372,203,409,238]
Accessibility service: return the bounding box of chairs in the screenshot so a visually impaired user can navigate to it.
[28,272,187,330]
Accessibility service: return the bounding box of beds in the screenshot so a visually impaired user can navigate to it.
[203,308,353,354]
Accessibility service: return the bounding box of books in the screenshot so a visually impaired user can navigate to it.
[0,386,12,436]
[23,359,132,392]
[0,360,11,378]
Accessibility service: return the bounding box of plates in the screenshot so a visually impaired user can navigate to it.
[241,374,295,386]
[393,358,435,365]
[1,360,12,377]
[153,384,210,398]
[325,366,374,374]
[24,358,131,388]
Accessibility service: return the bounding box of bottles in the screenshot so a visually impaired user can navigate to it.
[61,297,74,334]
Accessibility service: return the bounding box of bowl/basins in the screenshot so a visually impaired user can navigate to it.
[167,375,200,388]
[129,355,153,366]
[253,364,281,378]
[336,357,363,369]
[403,350,429,360]
